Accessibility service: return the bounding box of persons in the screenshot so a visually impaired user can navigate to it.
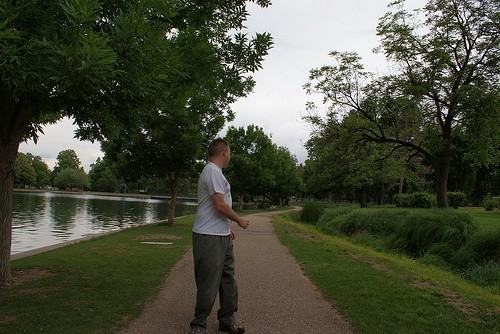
[189,139,249,334]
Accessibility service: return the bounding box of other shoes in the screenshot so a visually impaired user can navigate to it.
[219,317,245,334]
[191,325,207,334]
